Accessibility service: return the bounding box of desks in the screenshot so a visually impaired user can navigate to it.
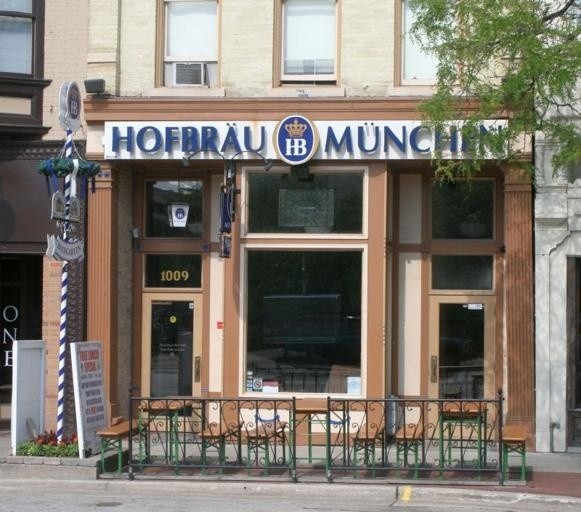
[438,401,487,469]
[286,399,342,467]
[138,401,193,465]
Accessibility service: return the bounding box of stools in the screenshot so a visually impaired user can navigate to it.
[96,419,146,474]
[502,425,527,481]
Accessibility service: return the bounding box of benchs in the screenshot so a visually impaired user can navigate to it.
[198,419,244,467]
[395,424,427,467]
[351,423,378,467]
[244,420,287,465]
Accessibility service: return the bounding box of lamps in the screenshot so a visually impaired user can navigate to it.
[181,150,272,222]
[166,179,192,228]
